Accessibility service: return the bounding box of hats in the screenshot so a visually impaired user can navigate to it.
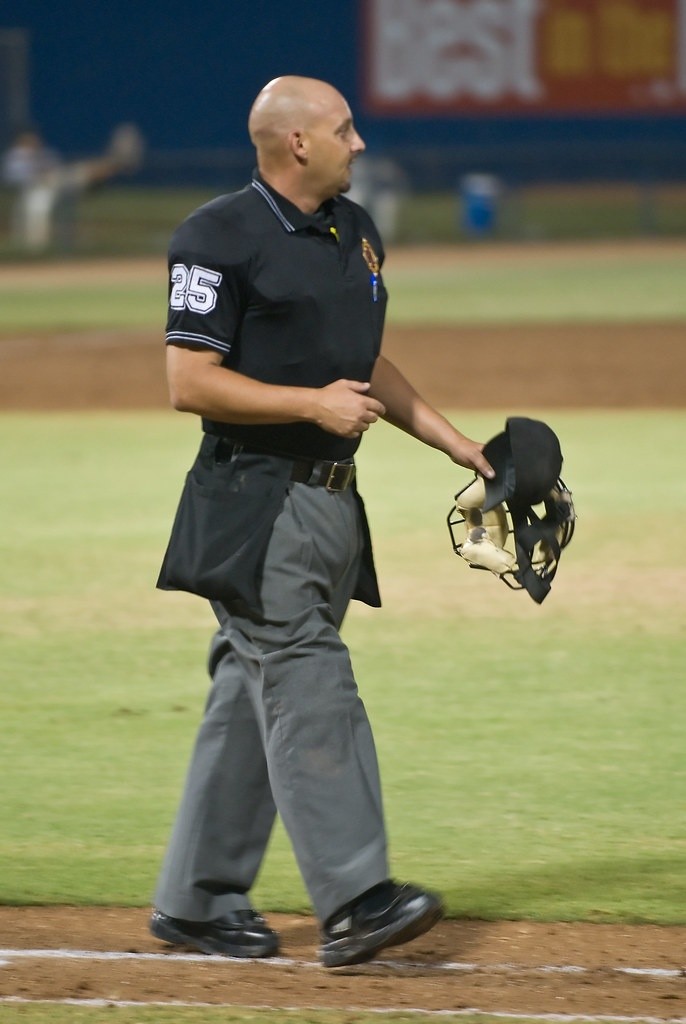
[481,417,563,513]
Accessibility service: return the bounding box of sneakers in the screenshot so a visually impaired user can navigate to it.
[148,906,277,958]
[319,882,444,967]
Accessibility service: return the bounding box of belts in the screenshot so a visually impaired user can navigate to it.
[198,436,357,492]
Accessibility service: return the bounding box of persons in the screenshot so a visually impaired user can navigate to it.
[148,76,496,969]
[8,125,144,262]
